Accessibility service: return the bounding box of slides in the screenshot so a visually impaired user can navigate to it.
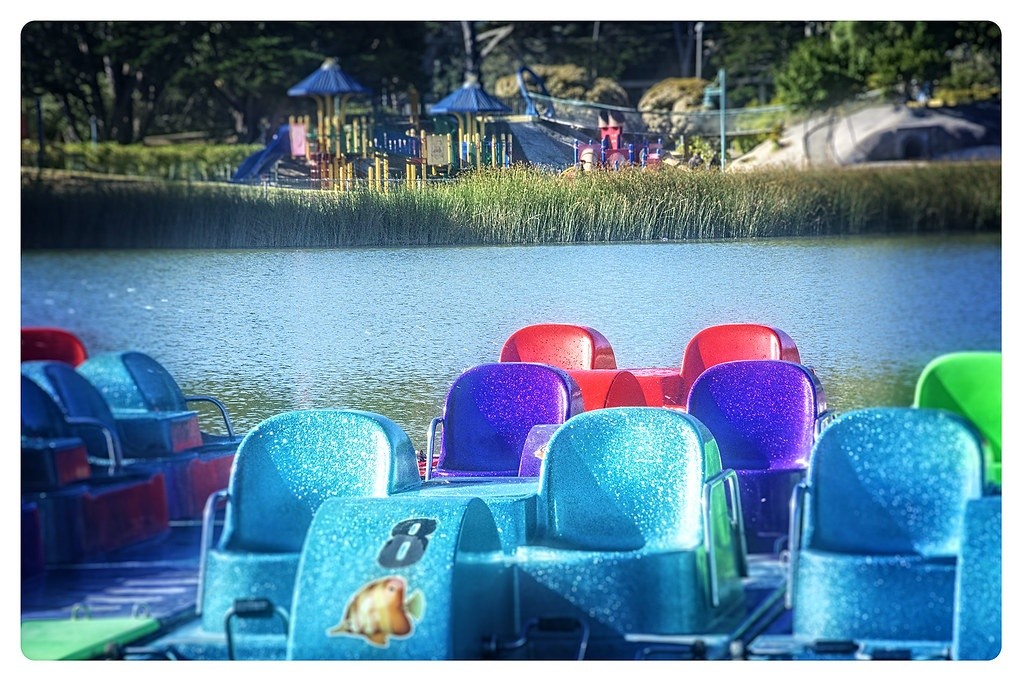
[581,149,598,172]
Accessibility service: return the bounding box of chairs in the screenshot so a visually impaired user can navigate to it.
[21,323,1002,661]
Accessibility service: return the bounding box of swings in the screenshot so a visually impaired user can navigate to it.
[230,122,291,183]
